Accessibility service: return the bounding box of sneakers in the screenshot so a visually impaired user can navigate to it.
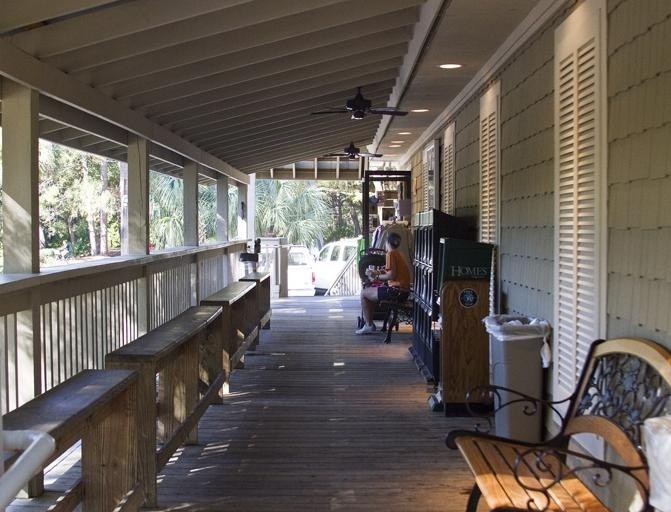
[356,324,376,334]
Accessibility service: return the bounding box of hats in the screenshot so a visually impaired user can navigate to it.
[387,233,401,246]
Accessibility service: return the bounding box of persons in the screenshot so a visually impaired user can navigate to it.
[355,232,411,335]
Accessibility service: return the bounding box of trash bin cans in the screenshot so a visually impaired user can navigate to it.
[484,315,551,444]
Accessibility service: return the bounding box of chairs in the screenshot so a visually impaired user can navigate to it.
[376,289,413,344]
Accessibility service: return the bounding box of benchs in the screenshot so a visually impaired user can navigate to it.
[444,337,670,512]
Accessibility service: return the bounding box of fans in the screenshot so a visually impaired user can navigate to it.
[310,87,409,121]
[321,140,383,162]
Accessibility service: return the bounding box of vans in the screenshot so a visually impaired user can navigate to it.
[315,237,363,294]
[259,245,317,298]
